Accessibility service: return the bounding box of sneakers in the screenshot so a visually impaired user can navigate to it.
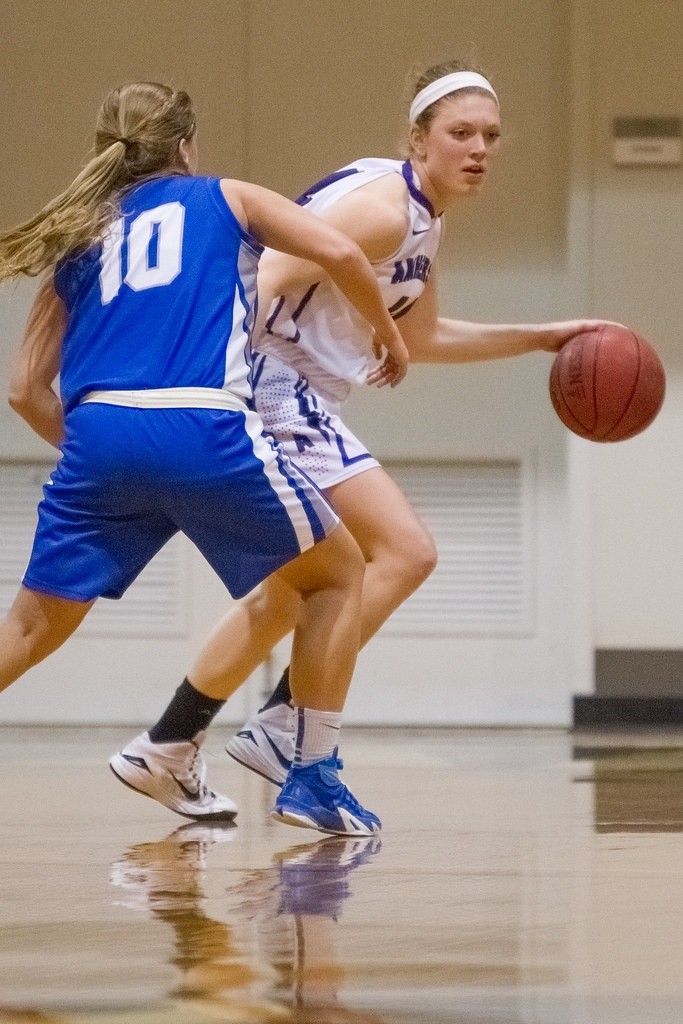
[225,703,295,788]
[110,729,238,822]
[110,820,238,894]
[270,746,383,835]
[274,835,381,917]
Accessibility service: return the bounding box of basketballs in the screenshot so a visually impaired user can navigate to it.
[548,322,669,445]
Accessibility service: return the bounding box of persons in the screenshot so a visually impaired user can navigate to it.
[0,81,409,838]
[109,59,628,824]
